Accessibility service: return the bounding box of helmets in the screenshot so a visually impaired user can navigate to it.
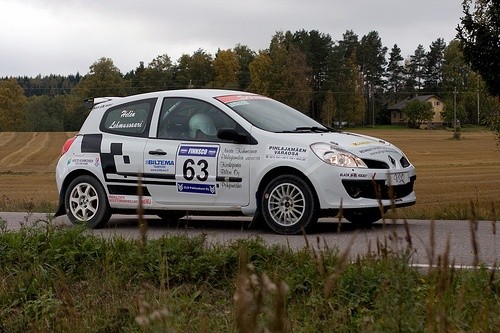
[188,114,217,138]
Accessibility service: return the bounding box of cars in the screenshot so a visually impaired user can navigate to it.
[53,89,416,239]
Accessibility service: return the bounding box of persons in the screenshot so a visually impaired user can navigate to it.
[177,113,222,141]
